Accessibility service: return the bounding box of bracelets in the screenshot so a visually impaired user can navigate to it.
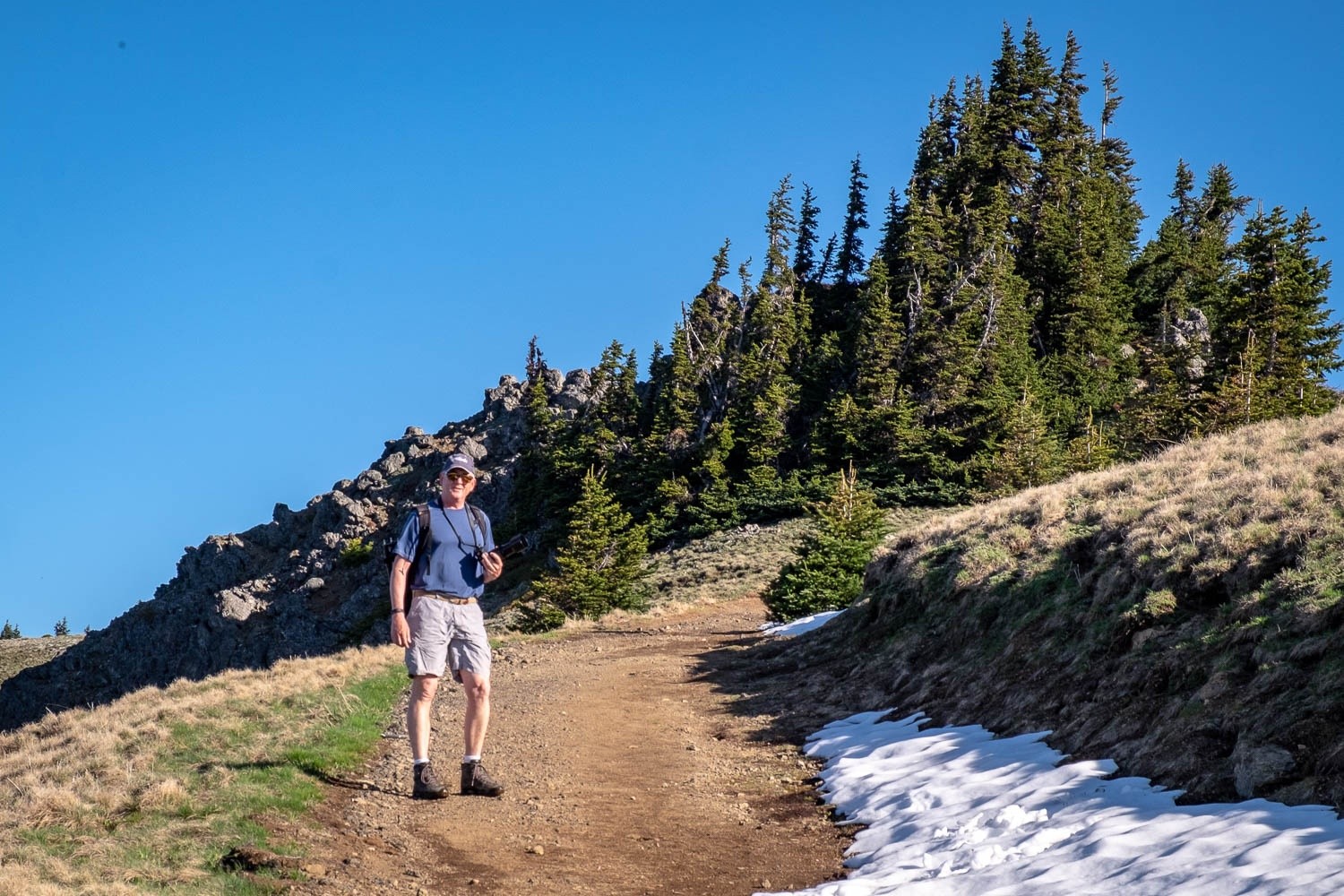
[390,608,405,614]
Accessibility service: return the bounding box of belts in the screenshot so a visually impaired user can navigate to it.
[414,590,476,605]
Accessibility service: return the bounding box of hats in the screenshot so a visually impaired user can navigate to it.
[443,454,475,478]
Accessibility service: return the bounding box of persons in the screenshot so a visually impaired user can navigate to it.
[390,454,505,799]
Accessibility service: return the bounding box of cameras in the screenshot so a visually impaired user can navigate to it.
[474,534,529,571]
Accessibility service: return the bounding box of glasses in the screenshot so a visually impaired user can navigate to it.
[447,472,473,482]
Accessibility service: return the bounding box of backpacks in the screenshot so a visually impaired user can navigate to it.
[382,504,487,618]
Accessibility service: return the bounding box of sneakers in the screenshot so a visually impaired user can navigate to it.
[460,759,505,796]
[412,762,449,798]
